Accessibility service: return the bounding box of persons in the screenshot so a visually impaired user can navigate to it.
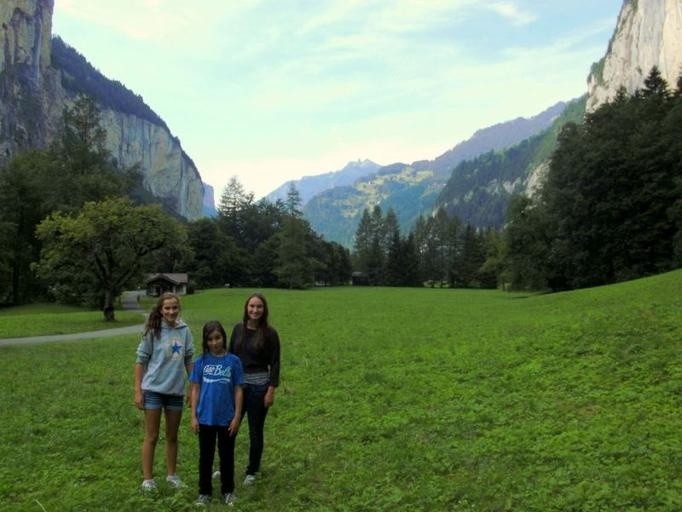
[188,321,246,506]
[132,292,196,490]
[211,293,281,488]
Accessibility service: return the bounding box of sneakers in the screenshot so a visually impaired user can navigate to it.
[142,471,256,506]
